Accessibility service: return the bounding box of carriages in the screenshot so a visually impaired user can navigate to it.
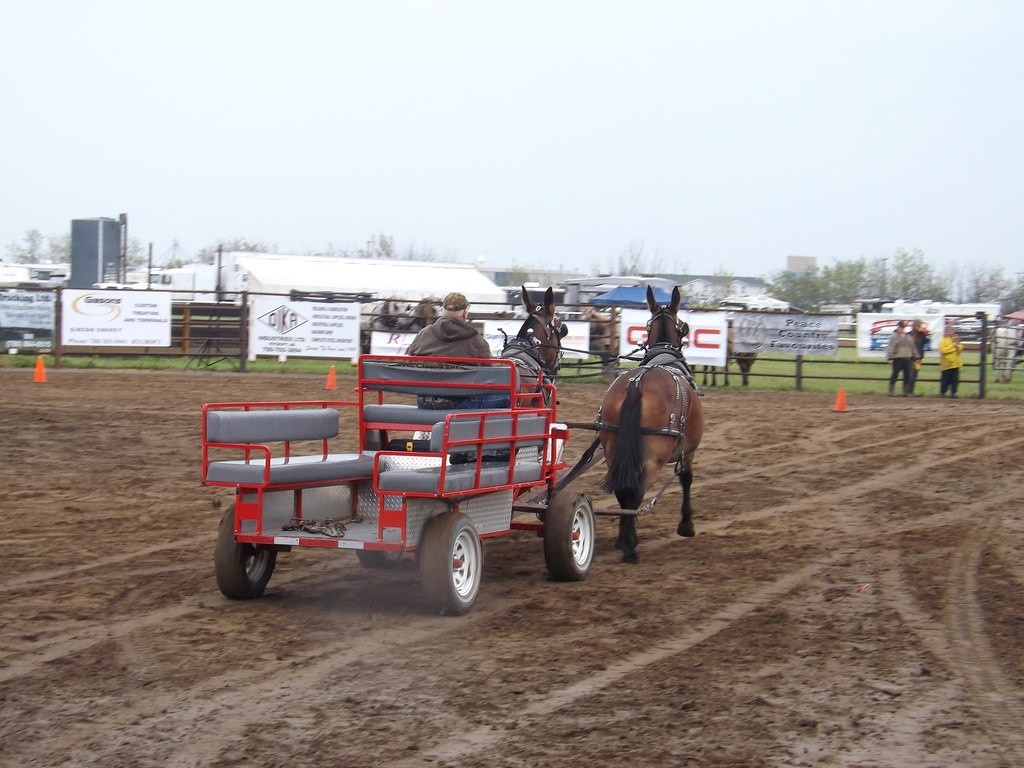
[192,282,704,618]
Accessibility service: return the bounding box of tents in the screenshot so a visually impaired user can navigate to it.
[587,284,689,310]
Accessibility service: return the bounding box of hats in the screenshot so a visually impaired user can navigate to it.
[898,322,906,328]
[443,292,468,311]
[914,320,924,325]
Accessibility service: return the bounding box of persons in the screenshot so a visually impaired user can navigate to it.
[907,320,930,397]
[408,292,509,410]
[886,319,921,398]
[939,324,963,398]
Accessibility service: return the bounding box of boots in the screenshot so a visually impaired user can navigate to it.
[888,382,894,396]
[903,384,911,397]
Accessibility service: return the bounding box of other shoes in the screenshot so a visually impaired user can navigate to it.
[951,393,959,398]
[939,392,946,398]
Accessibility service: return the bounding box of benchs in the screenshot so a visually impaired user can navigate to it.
[200,400,385,488]
[373,408,553,498]
[354,354,544,431]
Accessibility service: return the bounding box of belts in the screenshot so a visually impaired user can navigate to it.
[418,396,452,404]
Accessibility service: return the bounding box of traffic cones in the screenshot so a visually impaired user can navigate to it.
[31,355,49,382]
[322,364,340,390]
[831,387,850,414]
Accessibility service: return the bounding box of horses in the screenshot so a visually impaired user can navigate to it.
[412,296,445,332]
[593,284,706,565]
[360,293,412,346]
[500,285,569,414]
